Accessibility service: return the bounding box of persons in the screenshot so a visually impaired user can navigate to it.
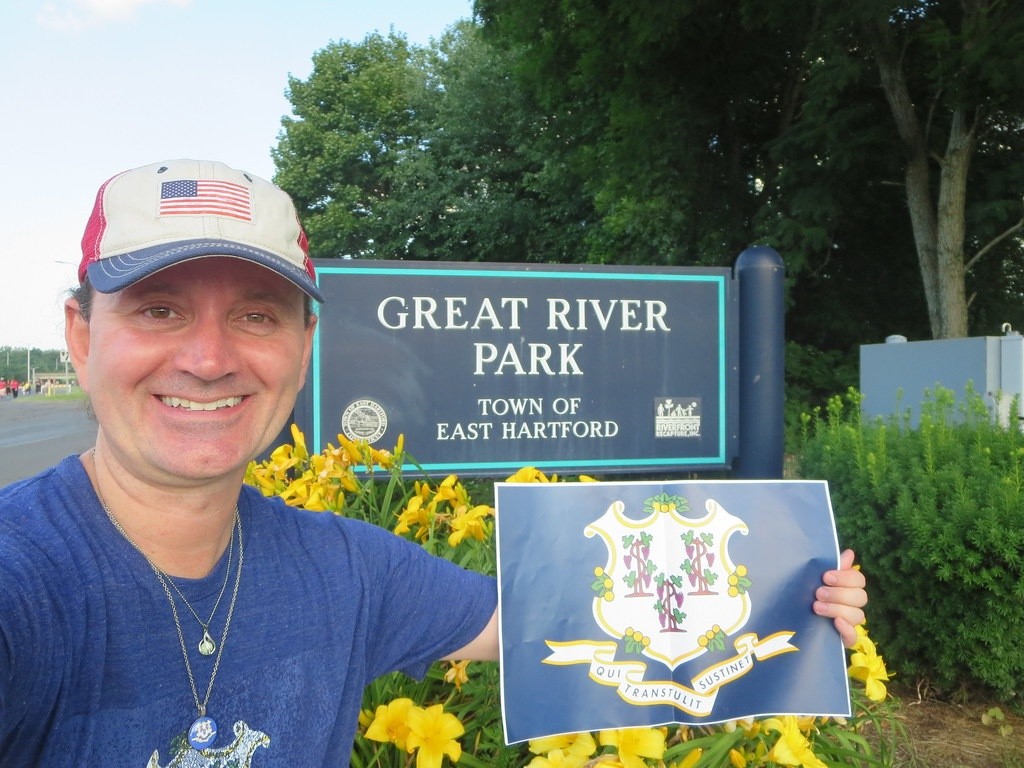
[1,157,868,768]
[0,375,42,399]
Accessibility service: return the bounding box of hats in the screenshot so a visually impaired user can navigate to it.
[79,158,326,305]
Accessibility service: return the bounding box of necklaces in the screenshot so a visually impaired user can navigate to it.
[104,502,244,752]
[93,452,236,657]
